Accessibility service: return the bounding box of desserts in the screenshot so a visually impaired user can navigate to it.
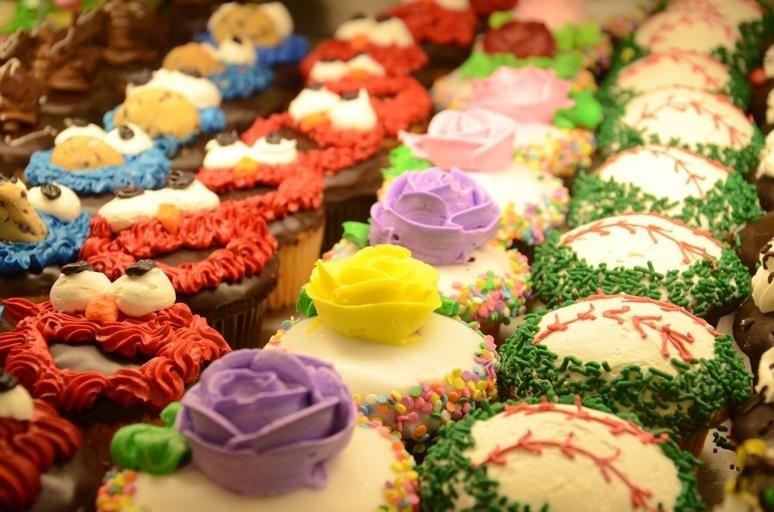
[0,0,774,511]
